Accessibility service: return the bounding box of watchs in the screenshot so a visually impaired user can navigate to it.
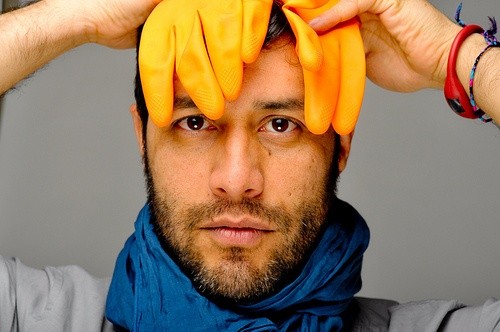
[444,25,486,120]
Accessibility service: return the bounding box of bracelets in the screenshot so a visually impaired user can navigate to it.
[453,3,499,124]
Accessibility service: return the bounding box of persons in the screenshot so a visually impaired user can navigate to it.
[1,0,500,331]
